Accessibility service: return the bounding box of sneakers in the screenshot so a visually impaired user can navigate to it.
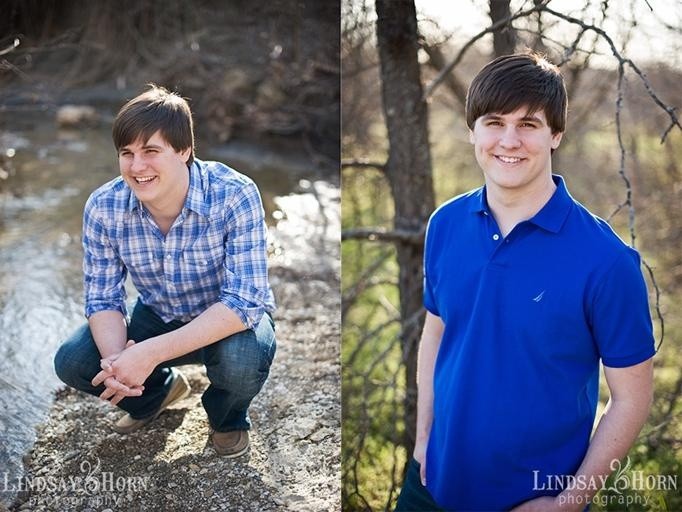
[113,366,191,435]
[211,430,250,459]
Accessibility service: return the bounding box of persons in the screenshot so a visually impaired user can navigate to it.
[52,81,276,461]
[389,53,658,511]
